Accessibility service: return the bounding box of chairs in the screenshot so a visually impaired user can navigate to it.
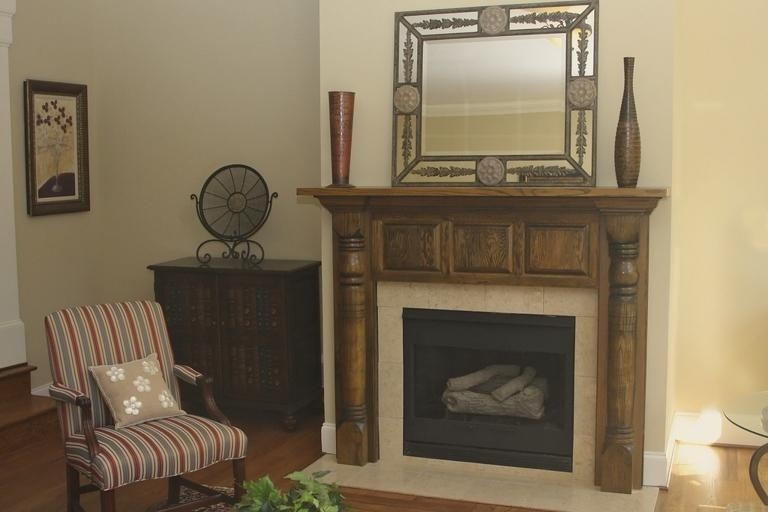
[44,299,247,512]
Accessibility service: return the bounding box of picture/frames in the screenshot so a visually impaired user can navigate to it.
[23,78,91,218]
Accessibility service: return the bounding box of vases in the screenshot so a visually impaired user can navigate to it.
[328,90,355,188]
[613,56,643,186]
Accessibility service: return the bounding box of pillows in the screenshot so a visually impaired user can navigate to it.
[85,352,187,431]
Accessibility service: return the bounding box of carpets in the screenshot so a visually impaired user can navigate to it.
[145,483,254,511]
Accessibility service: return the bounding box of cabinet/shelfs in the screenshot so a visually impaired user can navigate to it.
[146,255,322,433]
